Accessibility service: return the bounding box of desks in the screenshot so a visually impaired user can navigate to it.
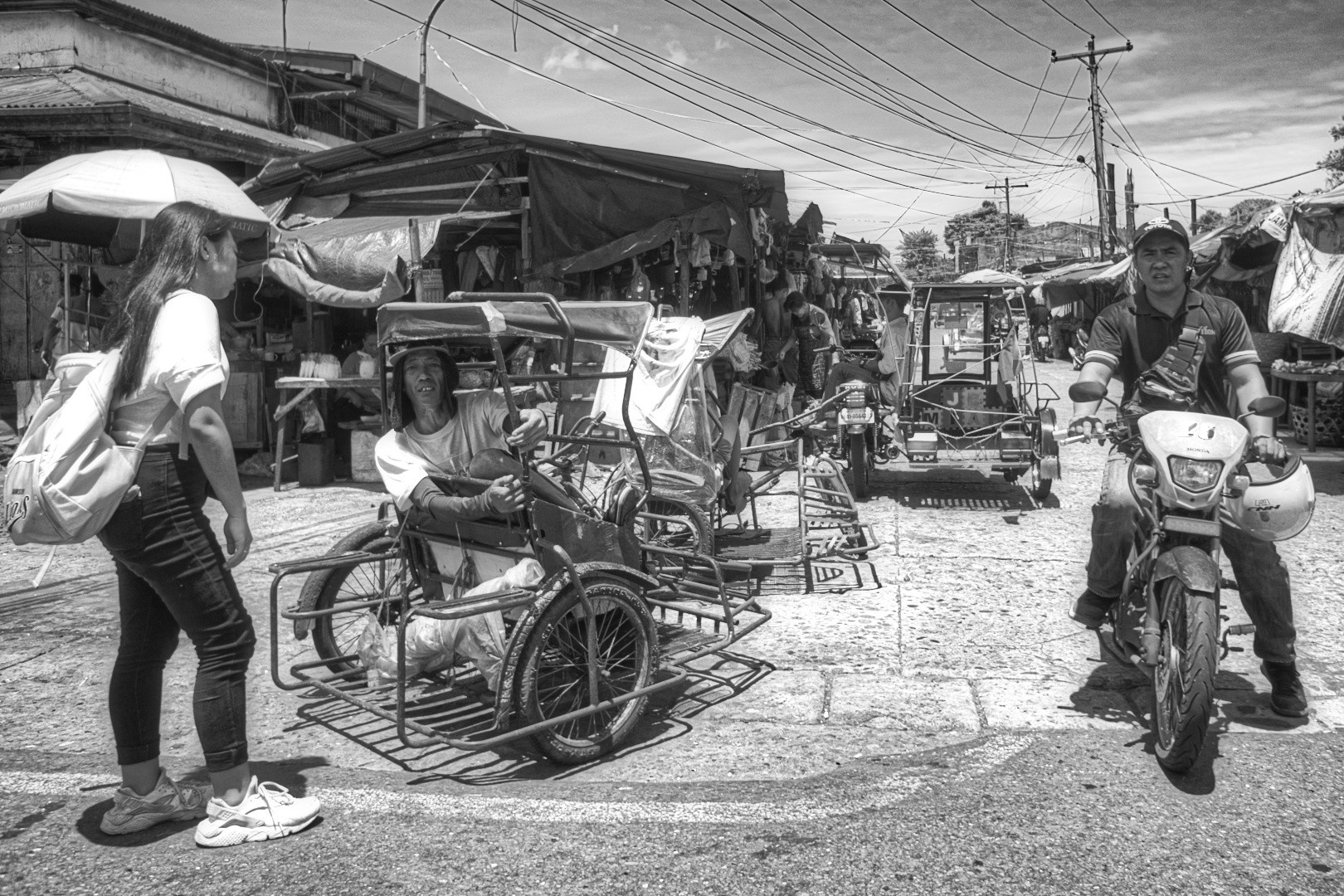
[1272,369,1344,450]
[270,375,379,492]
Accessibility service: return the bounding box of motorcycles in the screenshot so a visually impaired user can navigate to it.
[1049,381,1287,773]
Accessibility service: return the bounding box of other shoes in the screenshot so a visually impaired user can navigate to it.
[601,487,639,526]
[1022,354,1030,361]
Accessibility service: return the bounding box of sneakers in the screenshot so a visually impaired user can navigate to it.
[1067,588,1117,626]
[194,775,323,847]
[98,765,215,834]
[1261,658,1308,715]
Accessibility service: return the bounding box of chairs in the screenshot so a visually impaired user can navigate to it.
[401,497,641,615]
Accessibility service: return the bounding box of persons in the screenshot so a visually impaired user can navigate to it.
[1068,217,1309,717]
[1029,297,1054,351]
[41,273,109,380]
[100,200,323,849]
[775,291,840,458]
[373,339,636,535]
[809,283,915,437]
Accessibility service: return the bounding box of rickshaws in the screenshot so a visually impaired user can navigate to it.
[266,279,1063,764]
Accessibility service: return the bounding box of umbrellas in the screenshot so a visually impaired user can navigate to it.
[0,148,272,262]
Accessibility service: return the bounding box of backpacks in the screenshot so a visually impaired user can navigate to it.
[4,288,194,546]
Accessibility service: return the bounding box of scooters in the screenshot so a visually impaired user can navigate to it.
[1033,324,1050,362]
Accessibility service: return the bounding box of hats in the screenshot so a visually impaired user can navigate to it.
[869,284,910,302]
[1133,218,1189,251]
[389,340,452,366]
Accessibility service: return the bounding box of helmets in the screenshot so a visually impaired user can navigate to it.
[1225,454,1316,541]
[1035,297,1044,306]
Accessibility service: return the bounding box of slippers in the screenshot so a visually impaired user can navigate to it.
[809,420,838,437]
